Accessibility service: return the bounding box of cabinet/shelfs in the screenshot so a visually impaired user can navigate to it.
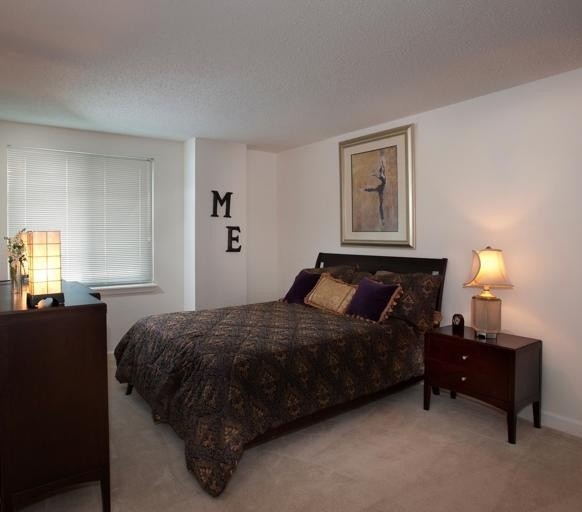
[1,280,111,512]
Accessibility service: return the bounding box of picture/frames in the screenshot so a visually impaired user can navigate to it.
[338,123,416,250]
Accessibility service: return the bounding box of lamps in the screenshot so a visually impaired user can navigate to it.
[462,246,513,339]
[20,230,65,308]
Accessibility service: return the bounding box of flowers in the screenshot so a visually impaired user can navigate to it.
[6,228,27,271]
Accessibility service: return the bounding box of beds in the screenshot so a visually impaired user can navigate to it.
[114,252,447,497]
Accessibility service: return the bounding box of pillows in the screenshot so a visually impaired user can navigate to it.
[280,263,444,329]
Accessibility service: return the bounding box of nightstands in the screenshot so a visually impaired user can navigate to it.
[421,324,542,444]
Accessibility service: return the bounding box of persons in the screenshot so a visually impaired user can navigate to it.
[359,160,386,229]
